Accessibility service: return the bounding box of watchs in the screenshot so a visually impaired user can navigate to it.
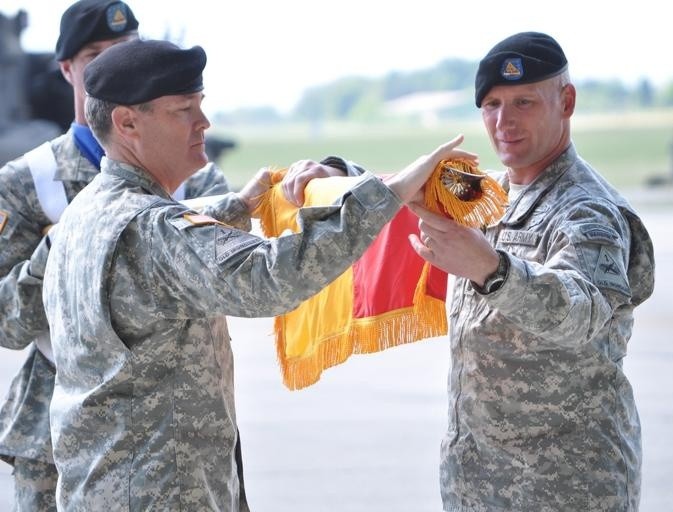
[470,249,511,294]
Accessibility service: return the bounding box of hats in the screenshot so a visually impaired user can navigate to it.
[54,0,139,64]
[475,31,569,108]
[82,38,207,107]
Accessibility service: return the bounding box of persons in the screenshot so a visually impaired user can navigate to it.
[401,34,655,512]
[0,0,231,512]
[41,40,478,511]
